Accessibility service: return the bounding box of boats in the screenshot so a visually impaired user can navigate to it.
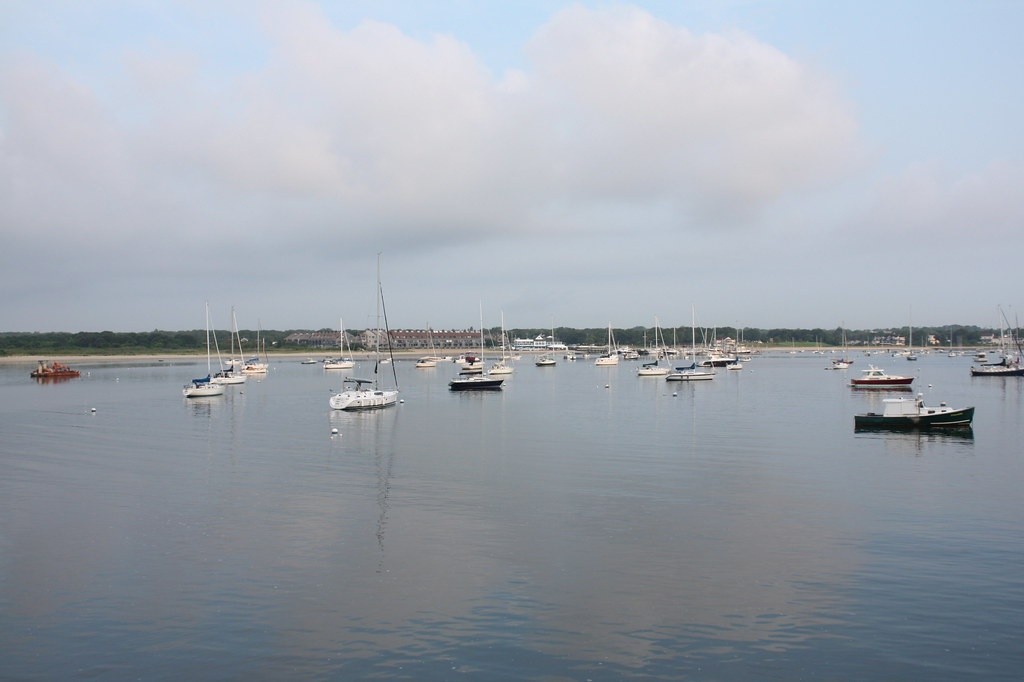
[455,354,480,364]
[31,360,81,378]
[459,365,484,378]
[415,360,436,368]
[534,356,557,366]
[379,358,392,364]
[224,358,243,366]
[301,358,317,364]
[246,355,259,364]
[321,356,350,362]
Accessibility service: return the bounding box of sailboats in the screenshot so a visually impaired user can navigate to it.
[239,318,269,374]
[448,300,504,390]
[328,250,400,410]
[594,321,619,365]
[210,304,247,385]
[487,310,513,375]
[323,317,355,369]
[183,299,226,396]
[420,330,452,361]
